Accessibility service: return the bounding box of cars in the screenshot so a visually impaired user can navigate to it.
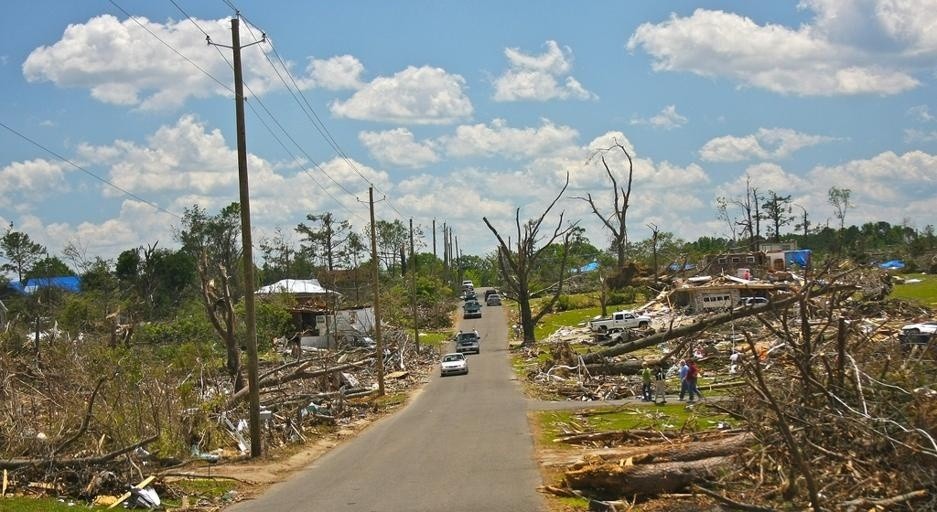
[902,322,936,335]
[746,297,769,308]
[461,279,501,319]
[440,353,468,377]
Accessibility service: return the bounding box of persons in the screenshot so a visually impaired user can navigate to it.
[685,356,706,404]
[743,270,751,282]
[641,361,654,402]
[651,364,668,404]
[677,357,693,402]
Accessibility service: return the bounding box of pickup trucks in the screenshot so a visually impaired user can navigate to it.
[453,328,480,354]
[590,311,651,333]
